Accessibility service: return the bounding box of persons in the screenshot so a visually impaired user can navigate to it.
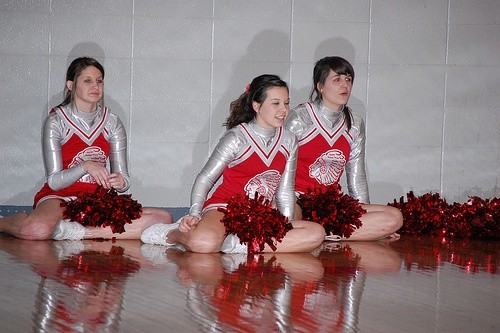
[140,74,326,253]
[0,57,173,240]
[282,56,403,241]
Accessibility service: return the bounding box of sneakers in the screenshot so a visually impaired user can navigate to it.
[140,223,180,247]
[220,233,251,256]
[52,219,86,241]
